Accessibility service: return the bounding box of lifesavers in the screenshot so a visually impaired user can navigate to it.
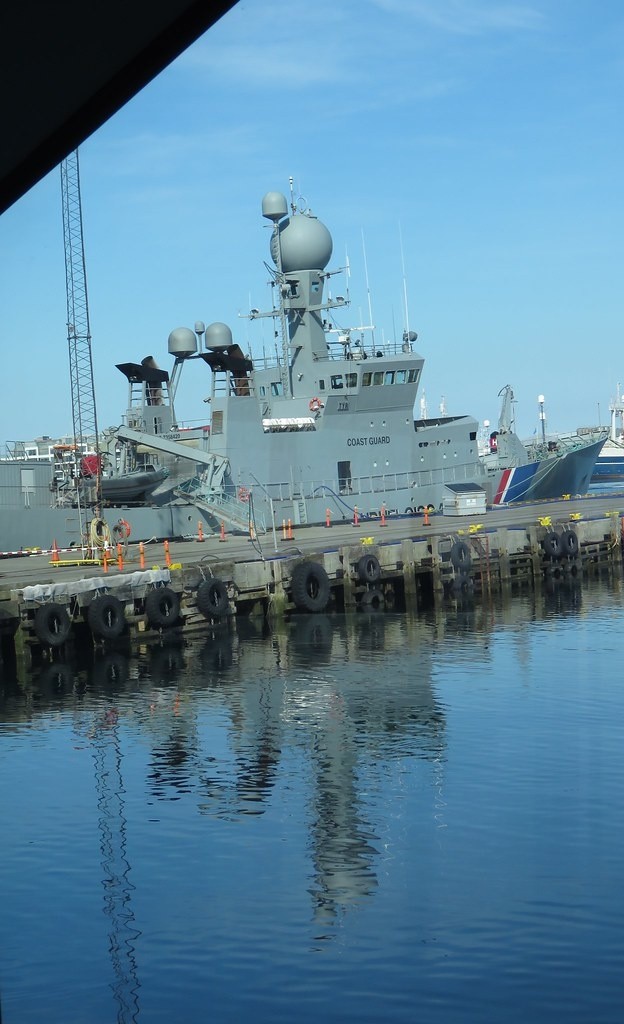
[87,594,125,640]
[147,646,187,688]
[451,575,474,591]
[292,614,333,668]
[361,589,384,604]
[37,660,75,703]
[117,521,131,538]
[290,561,331,613]
[196,578,229,619]
[199,638,233,673]
[449,541,471,570]
[88,649,131,695]
[238,487,249,502]
[542,529,583,576]
[308,397,321,411]
[357,554,381,585]
[144,587,181,629]
[33,603,72,648]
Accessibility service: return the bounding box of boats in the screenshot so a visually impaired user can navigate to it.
[0,174,615,560]
[523,382,624,481]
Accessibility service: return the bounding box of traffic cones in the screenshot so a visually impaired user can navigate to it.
[50,539,62,562]
